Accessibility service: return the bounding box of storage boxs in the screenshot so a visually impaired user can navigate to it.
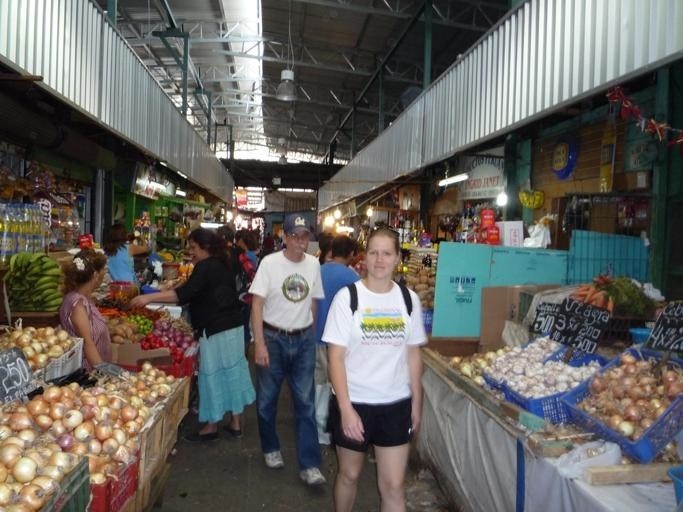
[474,283,563,352]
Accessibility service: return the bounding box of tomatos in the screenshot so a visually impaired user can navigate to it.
[137,333,194,376]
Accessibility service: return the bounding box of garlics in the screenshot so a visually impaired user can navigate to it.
[451,336,600,464]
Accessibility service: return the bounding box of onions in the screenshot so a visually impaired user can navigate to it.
[0,326,176,512]
[576,352,682,456]
[152,319,193,349]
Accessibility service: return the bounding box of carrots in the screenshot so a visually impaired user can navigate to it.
[96,306,127,318]
[569,286,614,313]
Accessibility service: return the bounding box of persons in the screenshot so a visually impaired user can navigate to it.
[319,226,428,512]
[60,248,113,373]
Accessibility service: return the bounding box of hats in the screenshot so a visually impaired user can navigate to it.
[282,213,312,238]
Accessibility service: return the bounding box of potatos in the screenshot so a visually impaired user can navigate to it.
[103,317,142,344]
[405,269,436,310]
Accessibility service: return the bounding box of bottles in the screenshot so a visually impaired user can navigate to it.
[394,243,410,274]
[396,217,428,245]
[597,102,616,194]
[143,219,149,238]
[462,199,473,243]
[0,200,52,264]
[417,251,432,275]
[134,222,141,238]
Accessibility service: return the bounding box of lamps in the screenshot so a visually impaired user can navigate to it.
[277,146,288,166]
[272,1,301,103]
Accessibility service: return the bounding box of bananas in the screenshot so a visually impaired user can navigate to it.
[2,252,64,312]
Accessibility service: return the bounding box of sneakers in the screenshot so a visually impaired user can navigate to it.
[298,466,327,487]
[221,425,244,439]
[183,430,223,443]
[263,450,285,470]
